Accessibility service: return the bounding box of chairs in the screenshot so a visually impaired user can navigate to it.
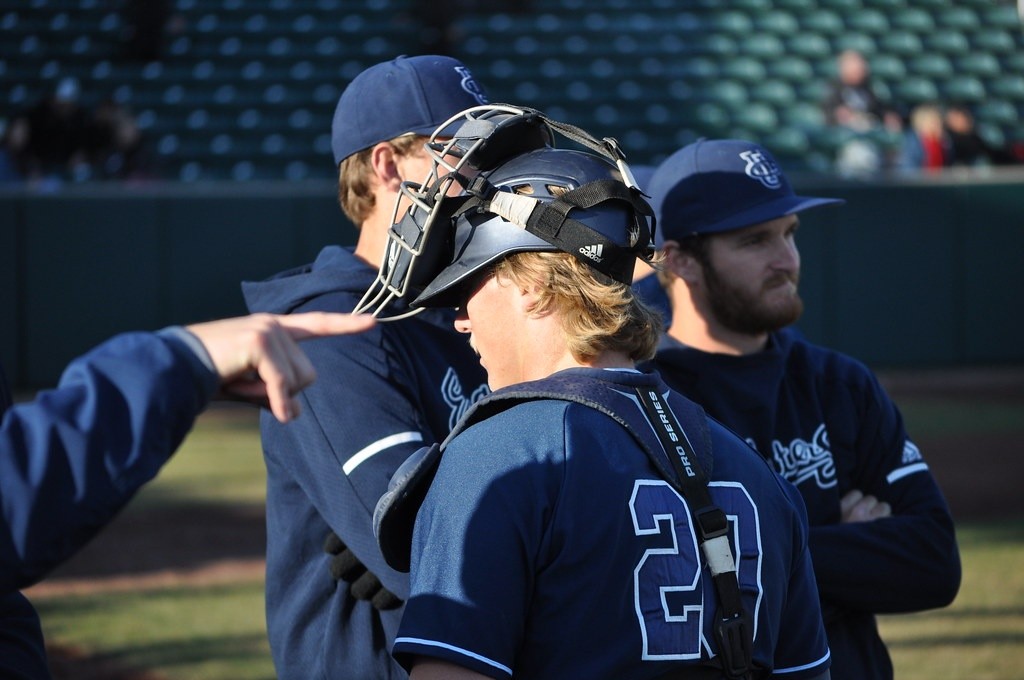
[0,0,1024,187]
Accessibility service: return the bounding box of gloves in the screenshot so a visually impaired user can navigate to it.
[326,533,400,609]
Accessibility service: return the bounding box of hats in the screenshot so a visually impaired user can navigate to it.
[331,54,491,167]
[646,135,845,252]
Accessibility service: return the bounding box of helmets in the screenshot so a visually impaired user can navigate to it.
[407,146,632,308]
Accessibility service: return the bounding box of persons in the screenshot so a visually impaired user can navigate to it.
[0,313,378,679]
[639,134,963,679]
[243,56,492,680]
[835,50,999,174]
[1,74,136,183]
[390,149,832,679]
